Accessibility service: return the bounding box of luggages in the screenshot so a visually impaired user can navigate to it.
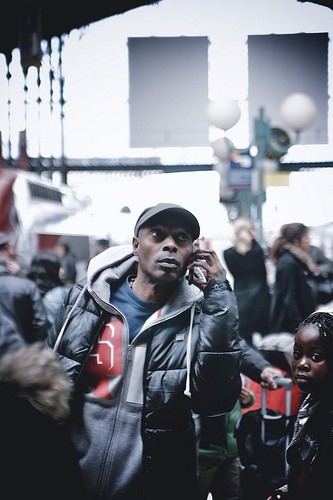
[236,378,292,500]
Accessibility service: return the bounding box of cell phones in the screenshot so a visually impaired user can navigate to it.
[189,244,196,285]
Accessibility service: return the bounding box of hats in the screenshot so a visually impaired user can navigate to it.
[134,203,200,240]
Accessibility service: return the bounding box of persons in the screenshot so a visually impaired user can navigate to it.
[224,217,333,341]
[192,335,284,500]
[266,310,333,500]
[46,202,241,500]
[0,235,110,356]
